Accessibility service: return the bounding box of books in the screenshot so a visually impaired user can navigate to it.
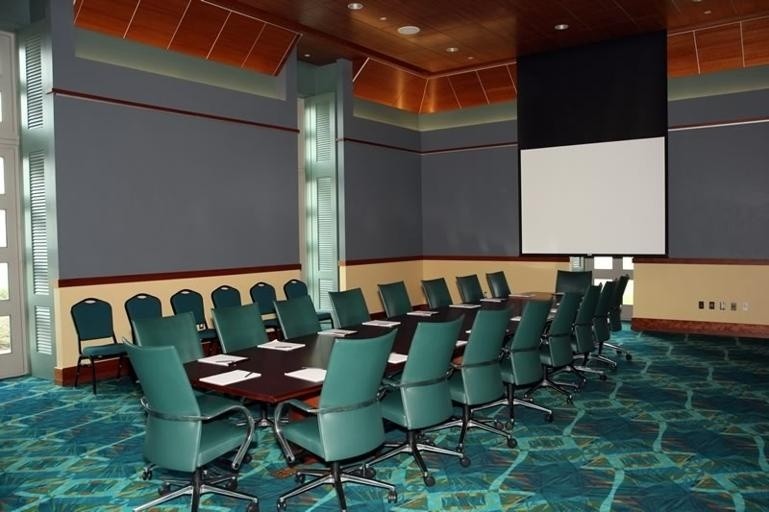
[448,302,482,310]
[361,318,402,330]
[509,293,537,298]
[256,335,308,355]
[406,308,440,319]
[510,315,522,323]
[197,369,263,388]
[316,326,358,338]
[547,290,565,323]
[197,351,249,369]
[387,351,410,367]
[465,329,472,334]
[456,339,469,348]
[480,297,507,304]
[284,364,328,384]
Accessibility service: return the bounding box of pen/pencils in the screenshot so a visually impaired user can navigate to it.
[215,361,230,362]
[276,346,292,348]
[245,372,252,377]
[334,332,345,334]
